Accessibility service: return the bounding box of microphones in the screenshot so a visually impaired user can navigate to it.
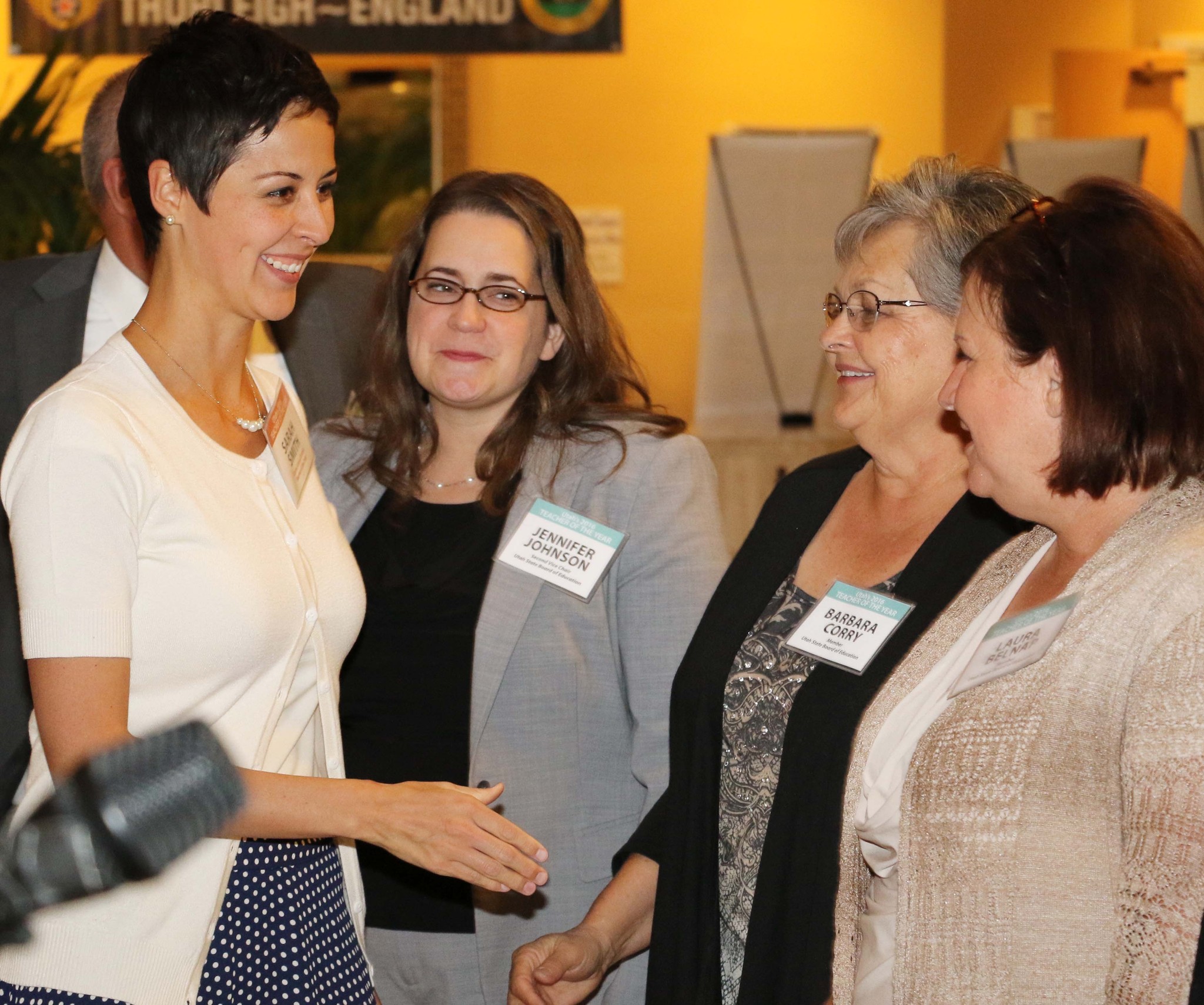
[0,721,245,947]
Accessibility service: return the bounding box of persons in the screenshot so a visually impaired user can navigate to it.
[508,155,1062,1005]
[831,175,1204,1005]
[306,169,730,1005]
[1,12,549,1005]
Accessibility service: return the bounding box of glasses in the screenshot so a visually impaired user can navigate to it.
[823,289,934,335]
[407,270,553,313]
[1012,197,1061,274]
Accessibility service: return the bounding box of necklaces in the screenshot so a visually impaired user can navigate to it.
[414,469,487,489]
[132,317,267,434]
